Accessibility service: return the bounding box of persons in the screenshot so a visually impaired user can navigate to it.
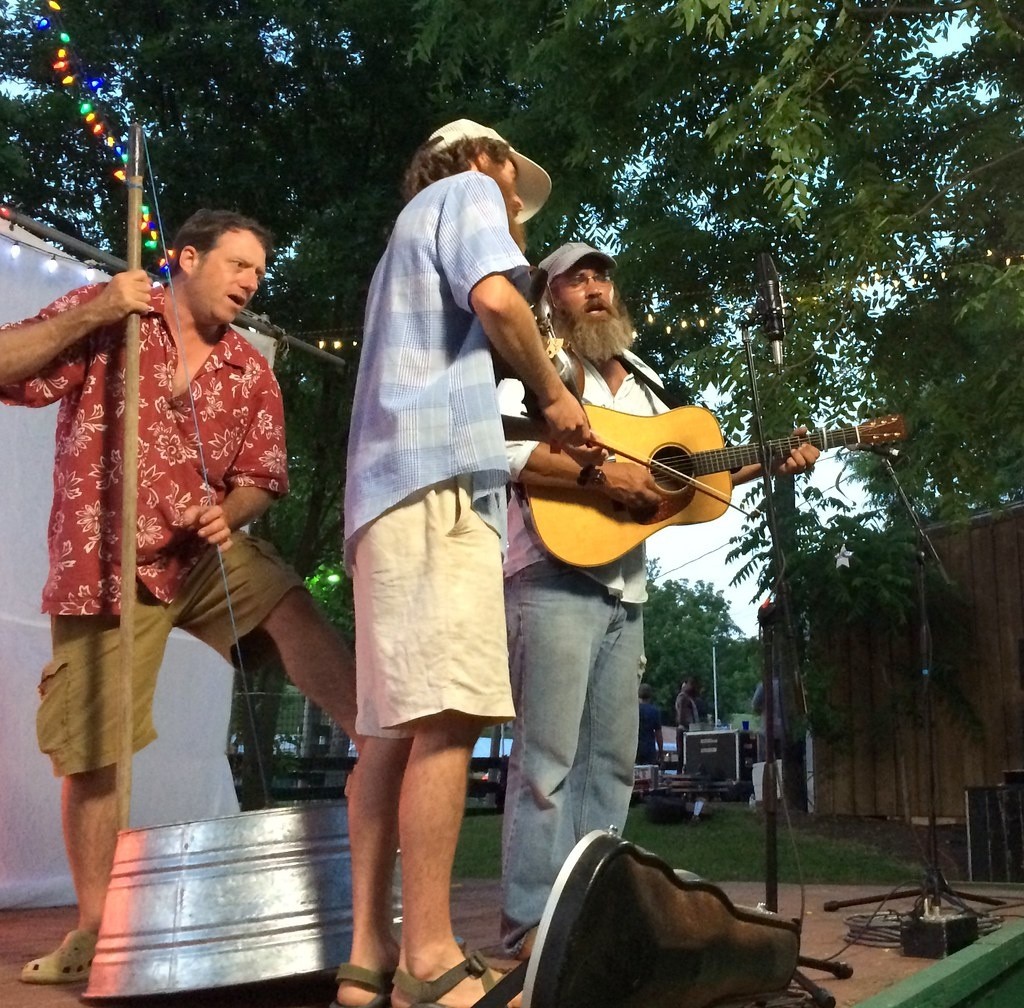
[329,118,820,1008]
[0,210,366,982]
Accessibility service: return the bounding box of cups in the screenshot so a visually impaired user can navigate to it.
[742,720,749,731]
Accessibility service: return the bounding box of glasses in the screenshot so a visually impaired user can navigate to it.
[565,271,612,289]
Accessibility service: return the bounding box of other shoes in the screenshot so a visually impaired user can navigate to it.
[516,927,538,957]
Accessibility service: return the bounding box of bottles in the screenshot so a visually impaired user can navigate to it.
[717,717,721,729]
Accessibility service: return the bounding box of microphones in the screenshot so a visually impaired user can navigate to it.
[846,443,906,458]
[756,253,783,375]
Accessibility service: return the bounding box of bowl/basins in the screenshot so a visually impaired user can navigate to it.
[81,800,401,997]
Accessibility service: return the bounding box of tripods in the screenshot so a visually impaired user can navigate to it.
[734,316,854,1008]
[823,457,1007,921]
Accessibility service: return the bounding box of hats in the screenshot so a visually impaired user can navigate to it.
[426,119,552,224]
[538,242,617,286]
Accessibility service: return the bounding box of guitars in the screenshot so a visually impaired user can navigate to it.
[519,402,910,570]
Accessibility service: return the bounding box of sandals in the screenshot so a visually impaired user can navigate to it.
[391,950,523,1008]
[328,941,468,1008]
[21,929,98,983]
